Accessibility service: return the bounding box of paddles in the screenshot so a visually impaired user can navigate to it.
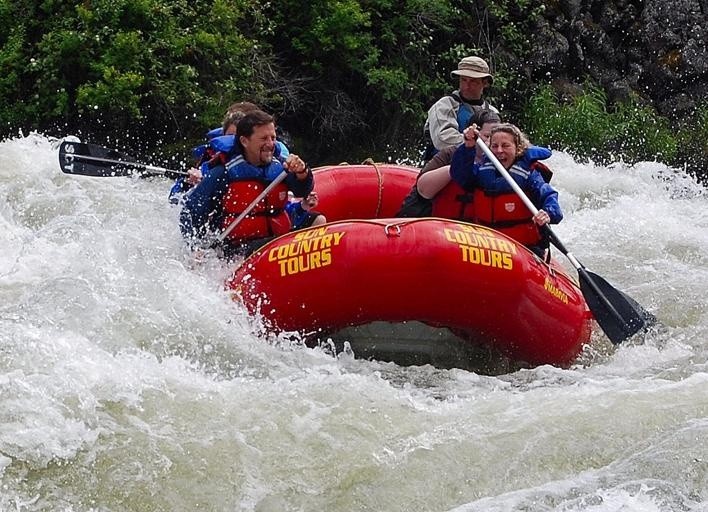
[540,222,658,335]
[472,133,647,345]
[58,140,205,178]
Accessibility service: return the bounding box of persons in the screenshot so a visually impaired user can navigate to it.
[394,56,574,263]
[169,103,326,275]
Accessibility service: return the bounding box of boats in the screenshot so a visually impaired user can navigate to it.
[226,164,592,367]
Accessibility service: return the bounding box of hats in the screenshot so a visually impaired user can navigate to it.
[450,56,493,85]
